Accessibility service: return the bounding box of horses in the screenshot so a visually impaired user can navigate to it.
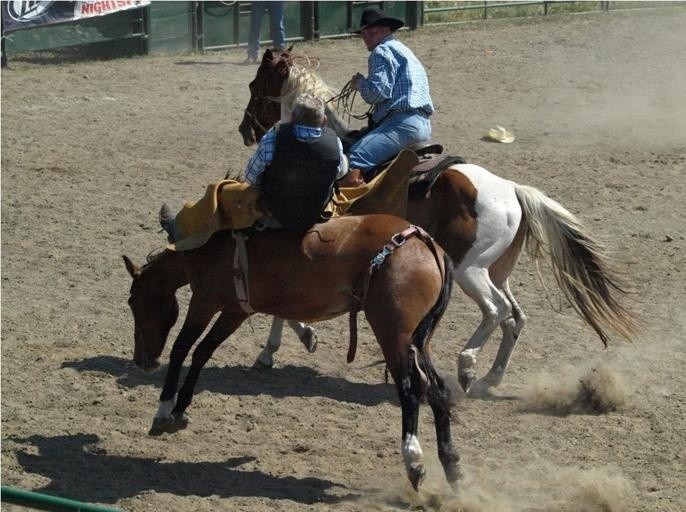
[239,42,647,397]
[122,166,468,495]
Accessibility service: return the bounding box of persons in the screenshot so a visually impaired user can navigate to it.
[160,93,348,245]
[242,1,286,65]
[343,7,434,174]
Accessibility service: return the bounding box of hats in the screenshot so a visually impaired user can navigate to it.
[487,125,515,144]
[349,6,405,32]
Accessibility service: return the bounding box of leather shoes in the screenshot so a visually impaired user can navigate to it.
[160,203,178,244]
